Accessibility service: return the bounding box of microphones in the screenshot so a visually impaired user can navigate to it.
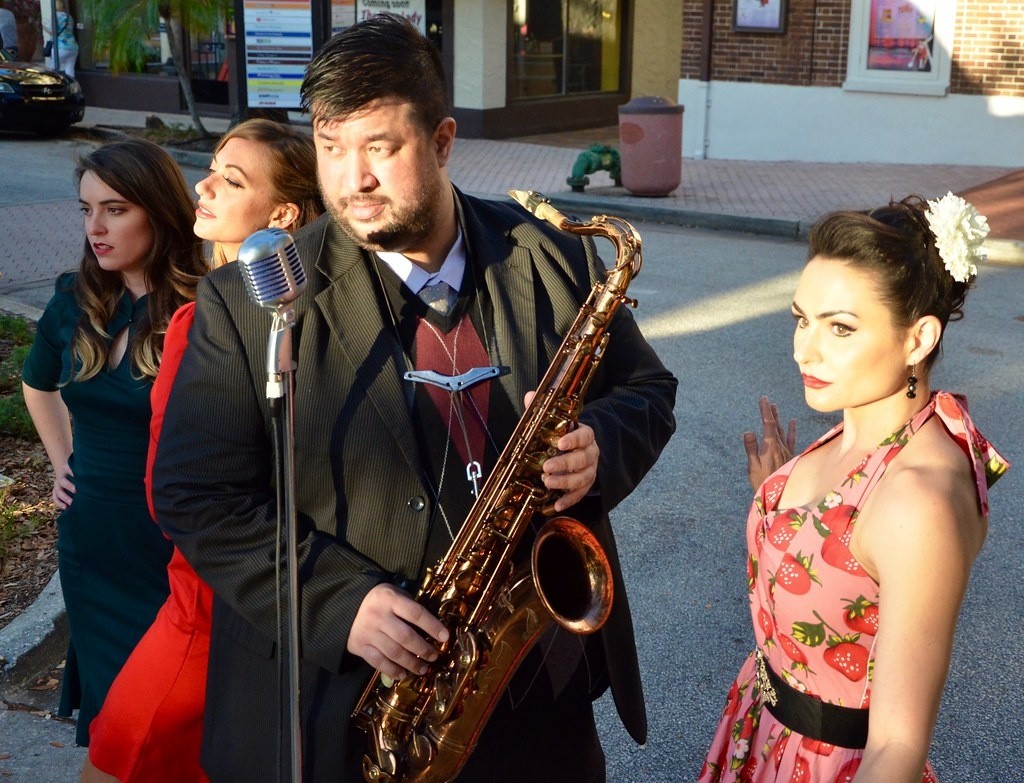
[237,227,307,422]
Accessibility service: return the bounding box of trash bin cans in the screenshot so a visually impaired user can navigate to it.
[617,94,685,197]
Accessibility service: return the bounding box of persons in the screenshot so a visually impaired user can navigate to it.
[80,119,330,783]
[151,13,680,783]
[696,191,1011,783]
[40,0,79,77]
[0,7,20,61]
[22,137,210,749]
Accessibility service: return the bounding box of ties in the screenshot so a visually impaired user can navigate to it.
[419,282,449,318]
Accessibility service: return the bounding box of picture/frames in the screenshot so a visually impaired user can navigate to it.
[732,0,789,35]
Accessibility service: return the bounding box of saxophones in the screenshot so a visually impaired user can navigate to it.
[350,188,645,783]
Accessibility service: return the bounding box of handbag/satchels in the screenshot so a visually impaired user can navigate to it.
[41,41,52,57]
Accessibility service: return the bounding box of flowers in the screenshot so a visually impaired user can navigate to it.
[923,189,989,282]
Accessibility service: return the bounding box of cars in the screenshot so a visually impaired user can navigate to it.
[0,44,86,137]
